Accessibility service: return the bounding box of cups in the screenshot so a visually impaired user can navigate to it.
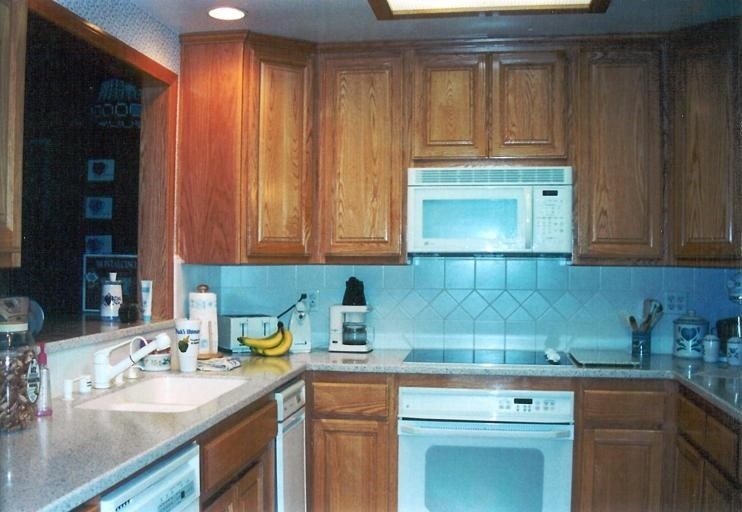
[98,280,125,321]
[175,318,202,370]
[703,333,721,362]
[726,336,742,365]
[631,329,651,358]
[671,309,709,358]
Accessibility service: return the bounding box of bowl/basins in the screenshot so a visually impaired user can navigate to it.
[140,353,171,371]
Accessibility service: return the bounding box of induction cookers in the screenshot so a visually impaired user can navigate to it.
[401,345,573,366]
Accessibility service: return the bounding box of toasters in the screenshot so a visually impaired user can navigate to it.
[217,314,279,354]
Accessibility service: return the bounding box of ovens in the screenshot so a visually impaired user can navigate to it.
[395,423,575,511]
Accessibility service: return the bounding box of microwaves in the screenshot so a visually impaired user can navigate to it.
[407,185,573,254]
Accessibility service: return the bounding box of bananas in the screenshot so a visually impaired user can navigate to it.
[237,321,293,357]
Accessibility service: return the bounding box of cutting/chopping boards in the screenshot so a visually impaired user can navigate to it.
[567,349,639,367]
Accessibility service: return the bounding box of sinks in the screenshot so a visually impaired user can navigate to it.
[71,375,253,413]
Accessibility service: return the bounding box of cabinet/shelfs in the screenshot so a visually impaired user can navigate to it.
[309,370,394,512]
[577,375,676,512]
[664,18,742,269]
[412,38,572,162]
[199,394,277,512]
[1,1,29,271]
[315,40,415,268]
[673,391,741,511]
[177,26,314,270]
[573,33,666,268]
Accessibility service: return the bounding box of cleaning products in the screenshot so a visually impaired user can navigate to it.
[35,342,53,417]
[188,284,219,355]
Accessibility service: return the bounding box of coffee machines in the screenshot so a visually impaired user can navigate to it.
[328,275,376,352]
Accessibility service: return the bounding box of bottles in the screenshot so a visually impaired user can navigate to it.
[714,294,741,362]
[0,321,39,432]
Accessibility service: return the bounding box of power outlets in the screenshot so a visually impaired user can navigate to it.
[664,287,688,315]
[295,290,318,313]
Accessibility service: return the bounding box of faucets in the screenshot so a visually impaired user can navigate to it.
[94,333,171,390]
